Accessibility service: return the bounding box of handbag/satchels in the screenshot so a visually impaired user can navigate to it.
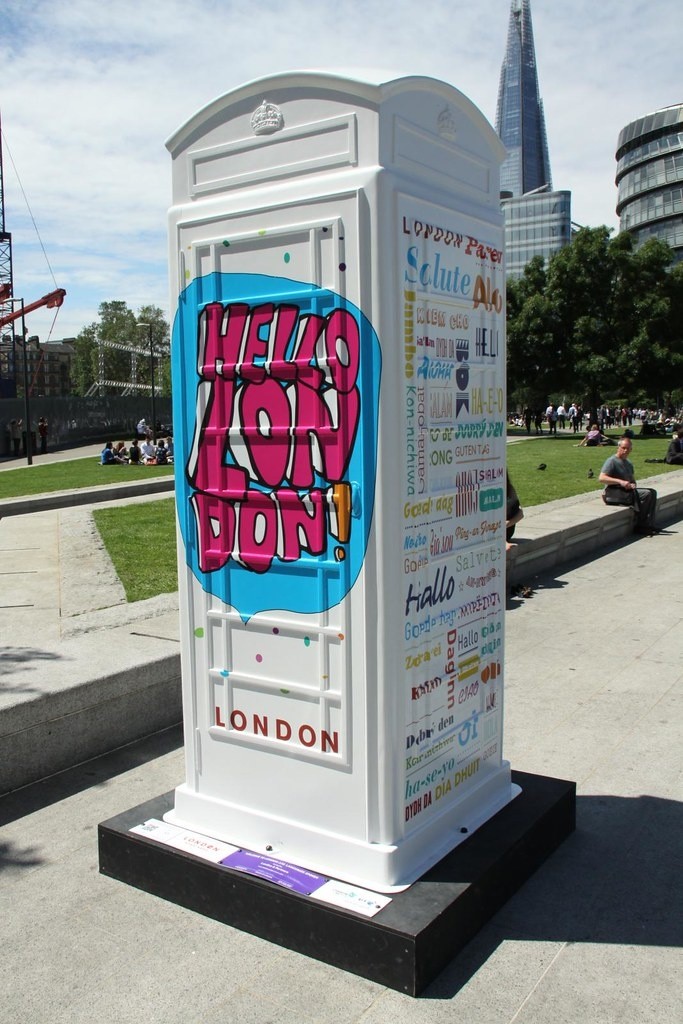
[605,484,635,506]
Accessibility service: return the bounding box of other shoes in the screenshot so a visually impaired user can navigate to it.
[511,584,532,598]
[633,524,661,535]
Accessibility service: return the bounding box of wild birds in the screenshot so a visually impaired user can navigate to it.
[537,463,547,471]
[587,469,594,478]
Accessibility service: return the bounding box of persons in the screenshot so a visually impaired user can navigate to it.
[666,424,683,464]
[508,401,683,447]
[506,466,524,553]
[137,418,173,438]
[598,438,662,536]
[11,416,49,456]
[101,436,174,465]
